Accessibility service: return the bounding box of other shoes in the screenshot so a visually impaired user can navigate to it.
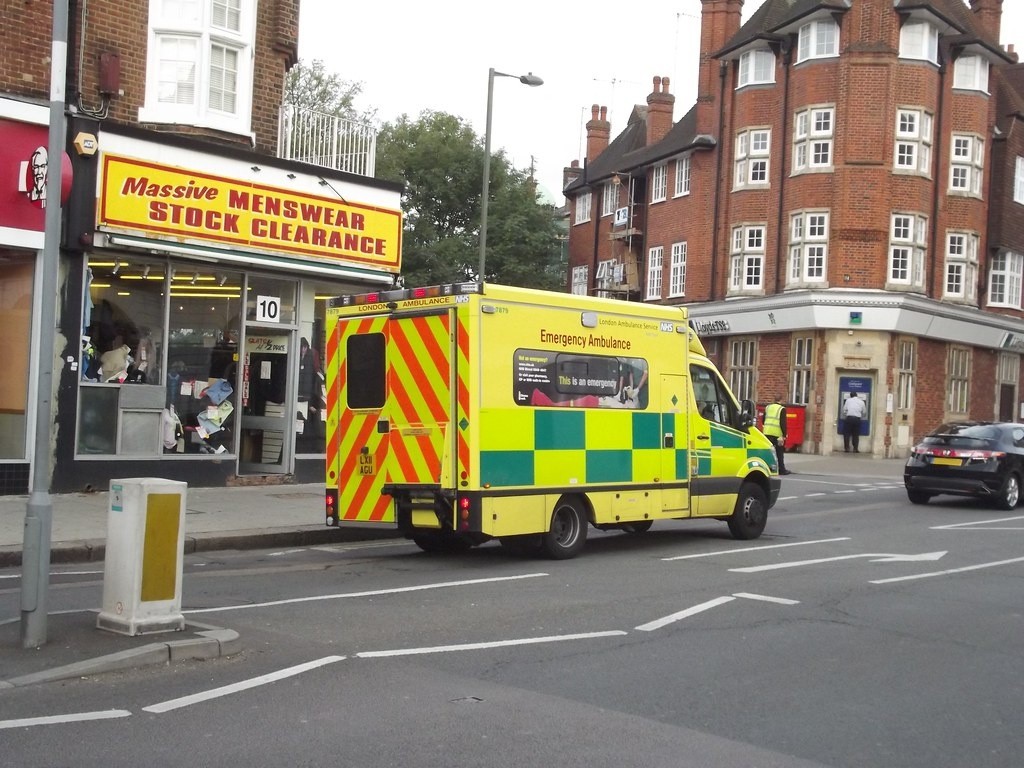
[854,449,859,453]
[780,470,790,475]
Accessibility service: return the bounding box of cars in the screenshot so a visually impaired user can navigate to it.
[904,420,1024,511]
[147,347,236,419]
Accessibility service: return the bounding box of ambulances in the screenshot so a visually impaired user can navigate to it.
[325,281,781,560]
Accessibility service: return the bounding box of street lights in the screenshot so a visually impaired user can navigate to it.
[478,68,544,282]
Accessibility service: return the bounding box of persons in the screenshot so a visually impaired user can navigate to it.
[132,326,156,382]
[843,391,866,453]
[298,337,321,403]
[761,396,790,475]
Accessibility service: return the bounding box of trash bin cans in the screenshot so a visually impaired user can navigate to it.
[92,476,190,638]
[755,402,807,453]
